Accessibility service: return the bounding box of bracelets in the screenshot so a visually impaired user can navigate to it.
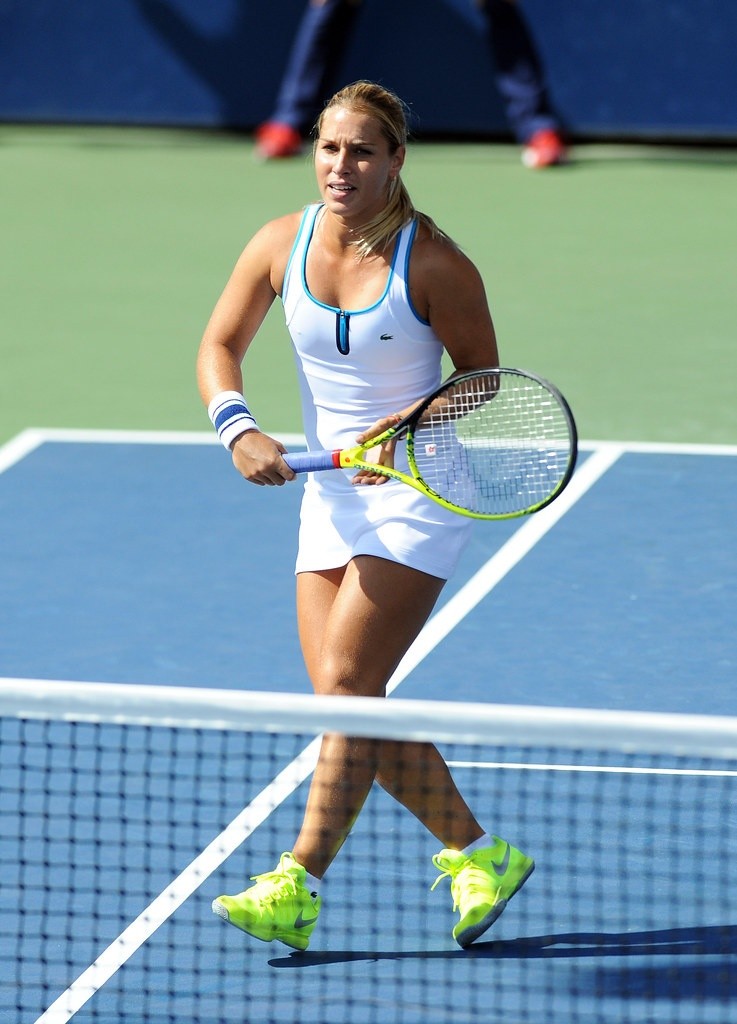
[207,390,260,449]
[392,413,403,422]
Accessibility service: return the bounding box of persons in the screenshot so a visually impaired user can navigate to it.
[197,81,535,949]
[258,0,569,166]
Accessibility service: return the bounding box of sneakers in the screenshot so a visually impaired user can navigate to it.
[257,124,301,157]
[523,129,562,168]
[429,834,534,949]
[212,852,320,951]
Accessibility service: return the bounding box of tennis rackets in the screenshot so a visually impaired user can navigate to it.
[280,366,583,522]
